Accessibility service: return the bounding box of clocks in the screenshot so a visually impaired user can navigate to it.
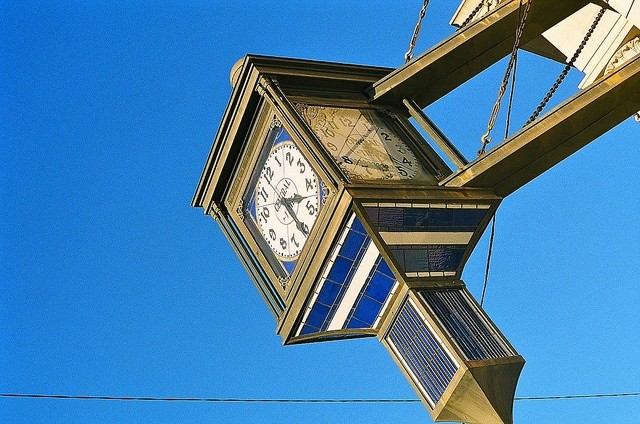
[291,95,434,188]
[221,97,330,311]
